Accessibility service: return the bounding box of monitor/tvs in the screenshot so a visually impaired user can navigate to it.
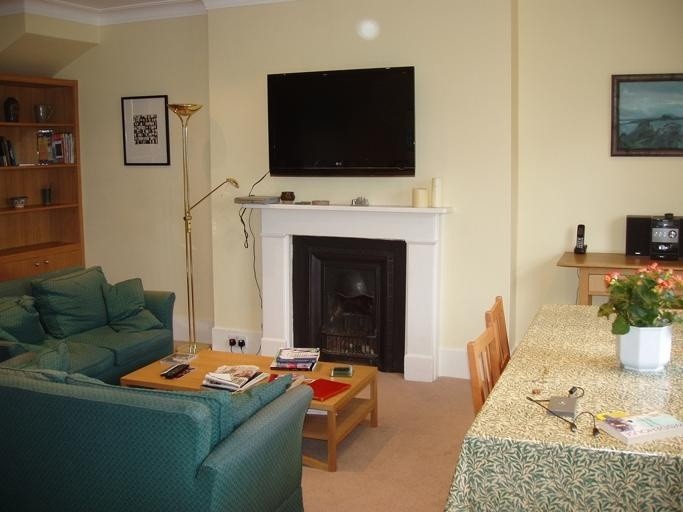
[267,66,415,177]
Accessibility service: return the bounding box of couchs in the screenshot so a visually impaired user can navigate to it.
[0,362,315,511]
[0,267,177,385]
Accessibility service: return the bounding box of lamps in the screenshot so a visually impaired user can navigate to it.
[167,103,240,354]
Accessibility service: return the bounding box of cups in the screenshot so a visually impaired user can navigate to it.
[431,176,445,207]
[33,103,55,123]
[411,187,429,208]
[41,186,55,207]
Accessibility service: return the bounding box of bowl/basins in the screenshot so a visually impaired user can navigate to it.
[7,196,28,209]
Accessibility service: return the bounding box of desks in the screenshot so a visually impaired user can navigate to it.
[555,251,683,310]
[439,302,682,511]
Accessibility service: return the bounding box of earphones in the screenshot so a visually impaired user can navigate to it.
[571,423,577,433]
[593,428,600,437]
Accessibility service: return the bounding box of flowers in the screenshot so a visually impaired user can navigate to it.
[595,259,683,335]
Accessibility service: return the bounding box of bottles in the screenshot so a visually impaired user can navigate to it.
[3,96,20,123]
[280,191,295,205]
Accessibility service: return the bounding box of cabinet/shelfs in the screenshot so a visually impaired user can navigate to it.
[0,72,86,282]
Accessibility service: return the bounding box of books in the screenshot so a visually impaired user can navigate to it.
[271,371,302,393]
[18,163,34,167]
[0,136,16,166]
[159,352,198,365]
[329,365,352,377]
[598,410,681,444]
[308,378,351,402]
[276,348,319,363]
[203,364,268,393]
[37,133,74,163]
[270,357,318,371]
[275,360,312,370]
[300,377,313,385]
[269,374,278,382]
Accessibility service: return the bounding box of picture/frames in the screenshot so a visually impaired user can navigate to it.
[608,72,682,159]
[119,94,170,168]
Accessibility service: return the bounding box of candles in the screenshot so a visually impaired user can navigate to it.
[411,186,428,208]
[430,176,443,208]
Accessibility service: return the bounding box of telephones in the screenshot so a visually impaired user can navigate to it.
[574,224,587,254]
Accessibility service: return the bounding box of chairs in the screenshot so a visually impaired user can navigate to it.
[463,319,501,418]
[483,294,511,374]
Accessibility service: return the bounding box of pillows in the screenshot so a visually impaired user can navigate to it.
[28,263,108,339]
[98,275,167,334]
[0,291,45,345]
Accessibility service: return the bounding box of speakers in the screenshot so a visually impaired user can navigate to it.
[626,217,651,256]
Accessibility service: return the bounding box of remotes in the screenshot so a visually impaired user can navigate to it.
[165,364,189,378]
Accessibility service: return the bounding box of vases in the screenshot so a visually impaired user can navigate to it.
[614,324,673,374]
